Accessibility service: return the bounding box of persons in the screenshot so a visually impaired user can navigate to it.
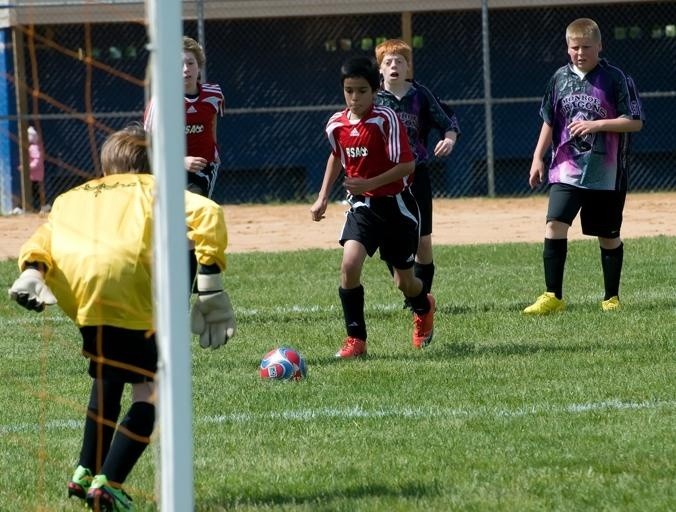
[309,56,436,360]
[5,123,242,510]
[364,40,464,299]
[516,15,644,319]
[141,38,228,297]
[15,125,47,210]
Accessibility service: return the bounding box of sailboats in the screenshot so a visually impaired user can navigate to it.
[260,349,306,382]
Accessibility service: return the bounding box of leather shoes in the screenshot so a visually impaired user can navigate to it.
[190,274,236,348]
[9,268,58,312]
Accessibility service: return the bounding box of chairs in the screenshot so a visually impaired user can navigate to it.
[523,292,564,313]
[335,336,366,357]
[68,465,132,512]
[413,293,435,348]
[602,295,619,310]
